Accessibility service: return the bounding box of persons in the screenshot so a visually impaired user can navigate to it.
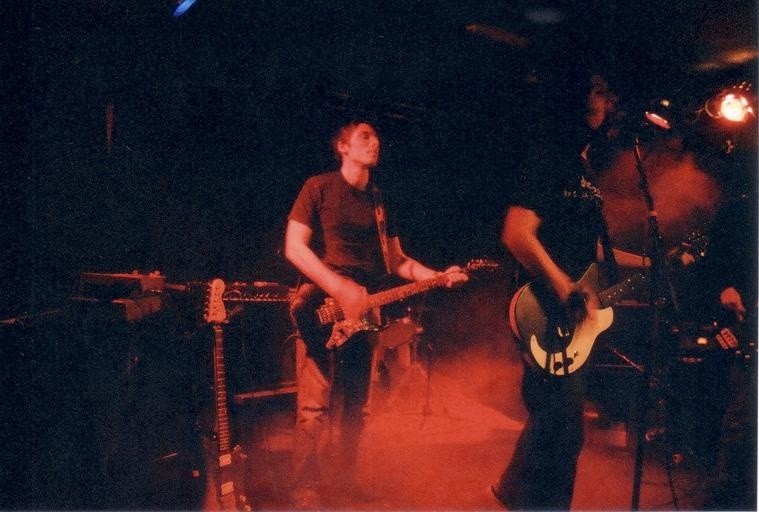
[285,120,468,512]
[489,68,695,512]
[644,241,746,466]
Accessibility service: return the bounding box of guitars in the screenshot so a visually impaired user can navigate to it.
[510,223,711,381]
[292,255,501,359]
[190,277,252,512]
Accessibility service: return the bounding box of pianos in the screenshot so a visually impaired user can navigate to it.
[175,281,301,303]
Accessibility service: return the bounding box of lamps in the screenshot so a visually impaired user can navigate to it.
[643,103,674,130]
[710,80,757,130]
